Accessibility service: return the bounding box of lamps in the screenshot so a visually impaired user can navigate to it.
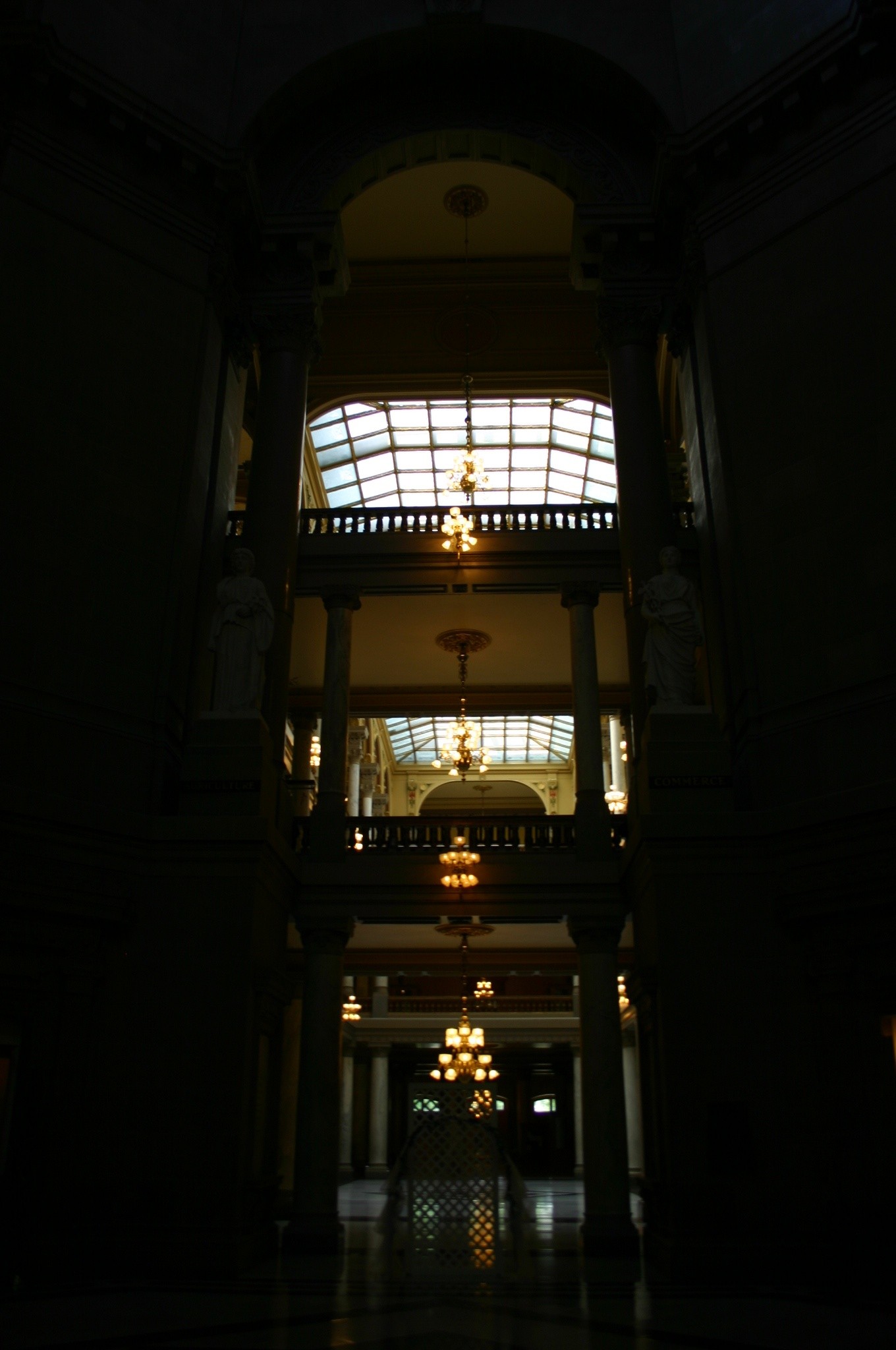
[431,629,492,783]
[441,507,478,560]
[474,973,495,998]
[443,183,489,502]
[311,736,321,766]
[341,996,362,1021]
[429,924,500,1085]
[439,836,481,888]
[605,784,627,814]
[619,741,627,761]
